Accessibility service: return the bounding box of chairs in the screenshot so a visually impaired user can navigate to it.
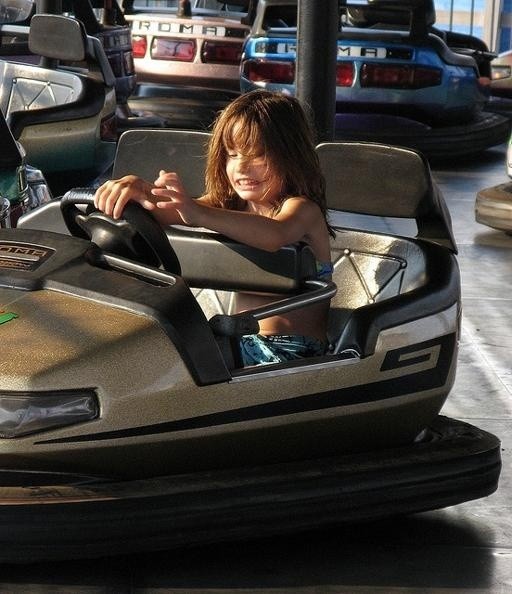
[1,12,95,168]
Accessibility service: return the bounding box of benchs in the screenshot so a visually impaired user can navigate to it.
[108,129,456,363]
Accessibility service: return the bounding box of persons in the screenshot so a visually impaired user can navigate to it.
[93,90,338,367]
